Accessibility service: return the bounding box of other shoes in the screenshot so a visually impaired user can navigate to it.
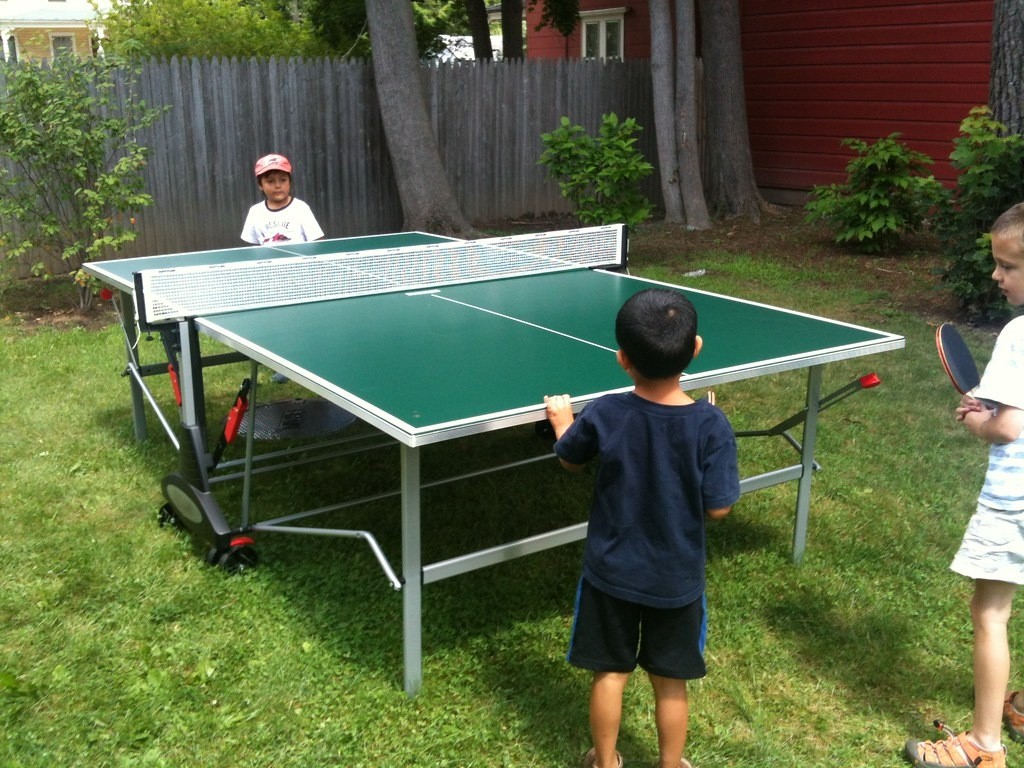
[270,373,289,384]
[585,743,623,768]
[657,757,692,768]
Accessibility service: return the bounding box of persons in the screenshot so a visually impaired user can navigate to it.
[904,202,1024,768]
[543,288,740,768]
[241,154,324,383]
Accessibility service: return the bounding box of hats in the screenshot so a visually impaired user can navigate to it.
[255,154,293,177]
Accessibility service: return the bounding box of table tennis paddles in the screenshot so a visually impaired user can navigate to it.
[706,386,720,413]
[935,321,981,396]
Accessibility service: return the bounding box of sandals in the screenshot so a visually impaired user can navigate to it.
[905,720,1007,768]
[1002,691,1024,743]
